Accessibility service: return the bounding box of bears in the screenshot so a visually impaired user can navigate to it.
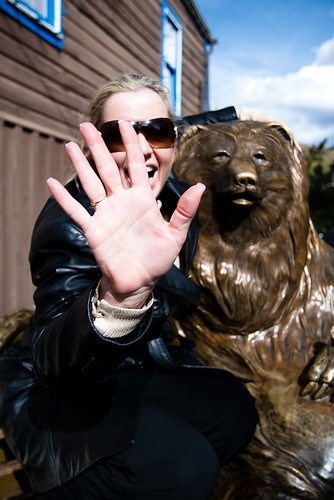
[0,119,334,500]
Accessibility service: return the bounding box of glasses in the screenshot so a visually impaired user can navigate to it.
[90,116,176,156]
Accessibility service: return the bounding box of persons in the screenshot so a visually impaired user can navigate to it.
[1,77,267,500]
